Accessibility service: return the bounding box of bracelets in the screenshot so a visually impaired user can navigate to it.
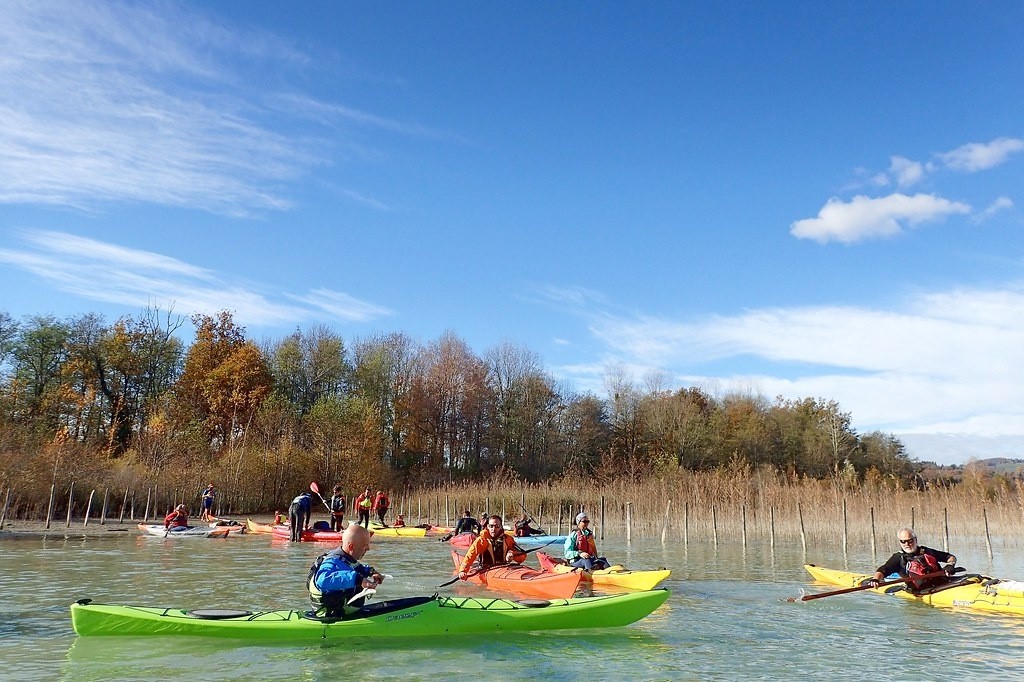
[869,579,879,583]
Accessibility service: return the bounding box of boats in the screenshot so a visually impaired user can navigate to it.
[804,564,1024,615]
[451,549,594,599]
[536,551,671,591]
[71,588,674,646]
[138,522,229,539]
[207,515,569,547]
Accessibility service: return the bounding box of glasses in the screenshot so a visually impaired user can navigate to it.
[900,536,916,544]
[583,520,590,523]
[489,524,500,528]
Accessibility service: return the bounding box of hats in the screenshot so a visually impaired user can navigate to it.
[575,513,586,526]
[208,484,214,489]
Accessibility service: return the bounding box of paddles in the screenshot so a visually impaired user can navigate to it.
[517,502,547,535]
[310,481,342,530]
[786,567,967,603]
[346,573,394,605]
[163,523,174,538]
[438,538,558,587]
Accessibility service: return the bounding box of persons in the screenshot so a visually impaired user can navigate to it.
[323,485,346,532]
[276,511,284,526]
[200,483,215,522]
[163,504,194,531]
[375,491,390,526]
[288,492,312,542]
[868,528,957,591]
[355,488,375,529]
[455,510,489,535]
[306,524,381,616]
[459,514,527,581]
[564,512,611,574]
[514,513,543,536]
